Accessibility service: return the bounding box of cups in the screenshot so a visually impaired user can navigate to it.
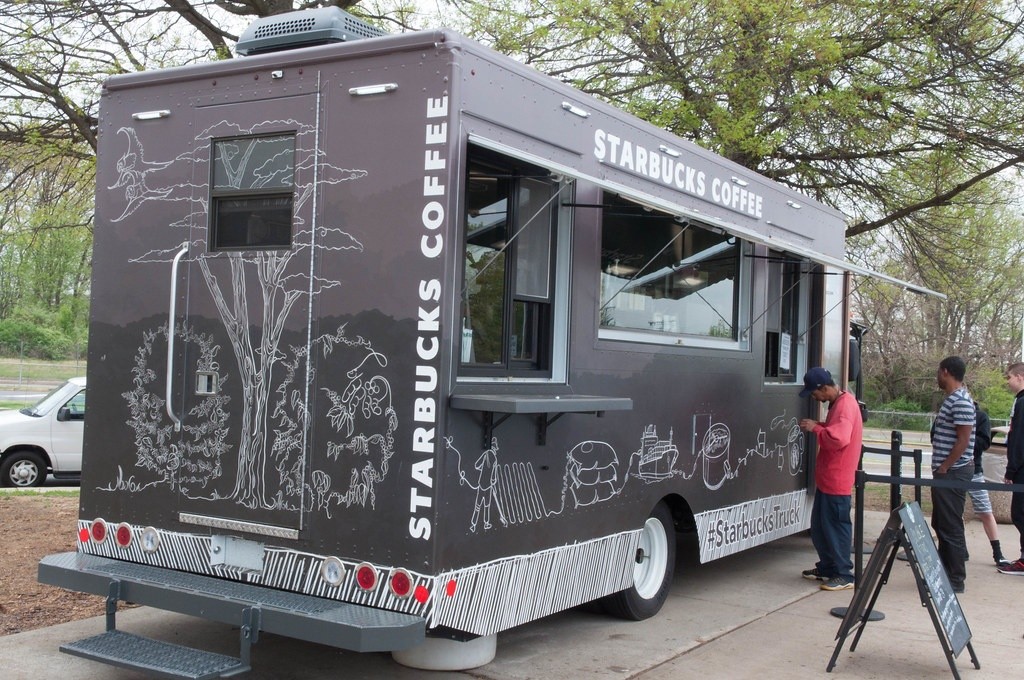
[461,329,474,362]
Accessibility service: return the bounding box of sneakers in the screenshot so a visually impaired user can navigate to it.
[802,568,830,581]
[820,576,854,590]
[997,558,1024,575]
[994,556,1011,565]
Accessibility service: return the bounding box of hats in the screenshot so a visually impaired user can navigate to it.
[799,368,832,398]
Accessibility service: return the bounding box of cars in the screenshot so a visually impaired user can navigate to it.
[1,376,88,487]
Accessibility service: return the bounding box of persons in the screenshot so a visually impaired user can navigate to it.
[997,362,1024,575]
[921,356,1012,594]
[799,367,863,591]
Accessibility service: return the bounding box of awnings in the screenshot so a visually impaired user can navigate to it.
[468,132,949,304]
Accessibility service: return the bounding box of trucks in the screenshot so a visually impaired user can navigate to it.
[38,6,948,680]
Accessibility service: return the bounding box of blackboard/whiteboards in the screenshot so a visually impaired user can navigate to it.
[834,500,974,660]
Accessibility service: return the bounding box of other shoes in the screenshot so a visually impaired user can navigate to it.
[953,582,964,593]
